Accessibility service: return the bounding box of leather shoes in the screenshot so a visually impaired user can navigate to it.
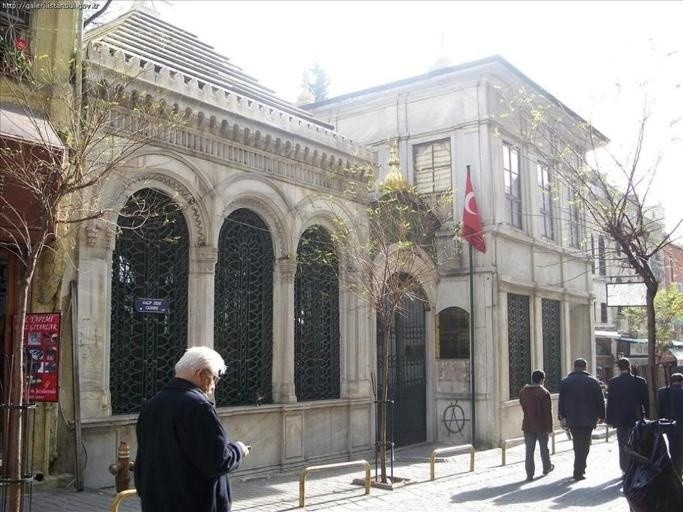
[543,464,554,474]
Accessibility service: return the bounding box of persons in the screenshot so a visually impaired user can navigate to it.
[556,358,604,481]
[605,356,648,477]
[131,345,250,511]
[656,372,682,478]
[518,370,555,481]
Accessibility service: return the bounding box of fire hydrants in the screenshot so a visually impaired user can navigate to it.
[106,440,136,494]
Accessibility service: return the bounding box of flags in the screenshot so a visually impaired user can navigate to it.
[461,171,486,254]
